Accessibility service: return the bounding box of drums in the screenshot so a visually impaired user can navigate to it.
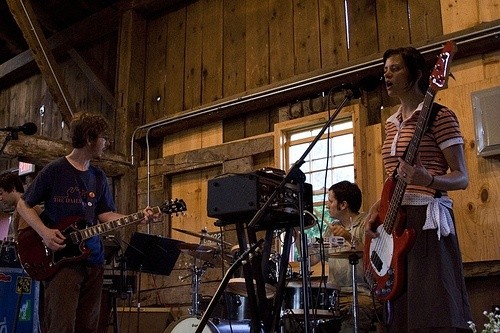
[164,285,342,333]
[227,243,282,298]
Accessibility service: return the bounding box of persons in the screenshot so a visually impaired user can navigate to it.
[364,47,470,333]
[0,170,42,248]
[293,181,372,311]
[16,111,160,333]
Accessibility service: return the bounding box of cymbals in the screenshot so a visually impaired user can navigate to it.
[172,228,232,247]
[326,250,364,260]
[176,243,235,265]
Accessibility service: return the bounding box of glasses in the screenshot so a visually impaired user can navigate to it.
[96,136,110,141]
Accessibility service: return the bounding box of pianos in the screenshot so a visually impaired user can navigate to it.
[101,274,137,333]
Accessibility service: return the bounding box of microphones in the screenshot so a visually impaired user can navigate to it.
[334,74,380,90]
[0,122,37,135]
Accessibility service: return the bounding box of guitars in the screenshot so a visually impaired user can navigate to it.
[13,197,187,280]
[363,40,457,302]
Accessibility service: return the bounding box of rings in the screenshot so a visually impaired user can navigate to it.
[403,173,407,177]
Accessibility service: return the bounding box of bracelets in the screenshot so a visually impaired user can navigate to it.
[426,175,434,188]
